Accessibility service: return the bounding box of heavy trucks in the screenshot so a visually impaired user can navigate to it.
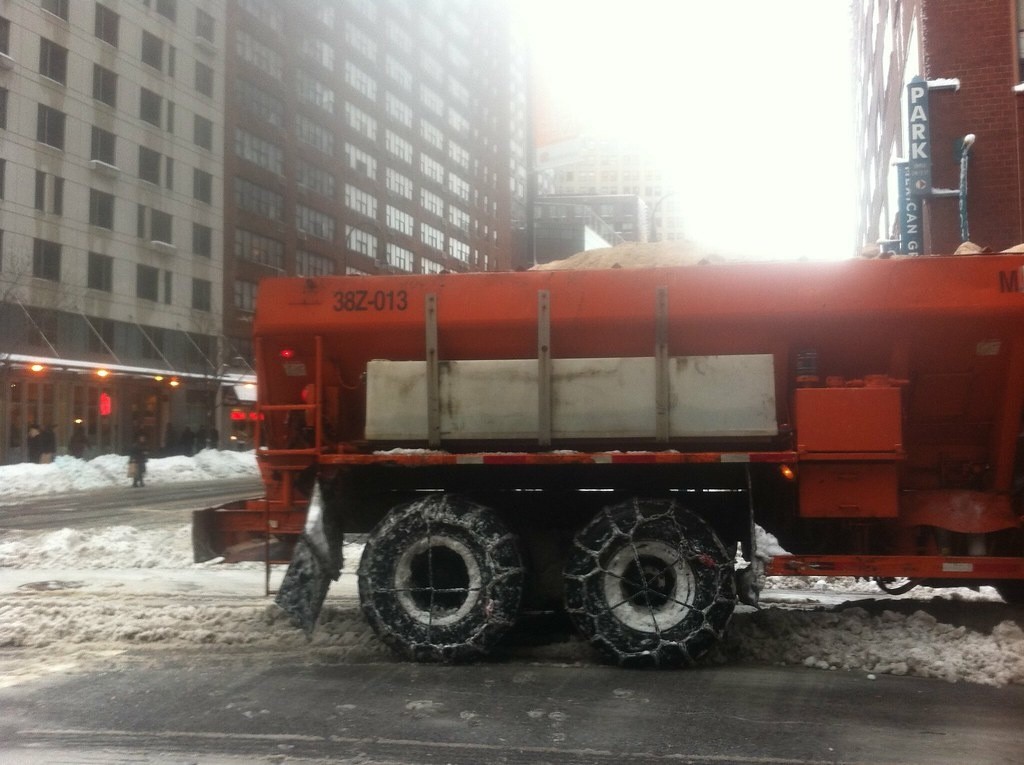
[189,258,1024,674]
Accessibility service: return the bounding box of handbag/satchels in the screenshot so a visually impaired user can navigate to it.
[128,460,137,478]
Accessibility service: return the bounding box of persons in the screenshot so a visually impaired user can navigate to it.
[210,423,219,449]
[26,424,56,463]
[129,433,150,487]
[179,426,197,457]
[165,422,178,457]
[197,425,208,454]
[70,426,92,459]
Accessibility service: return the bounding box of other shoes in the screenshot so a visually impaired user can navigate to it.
[133,484,138,487]
[141,484,145,486]
